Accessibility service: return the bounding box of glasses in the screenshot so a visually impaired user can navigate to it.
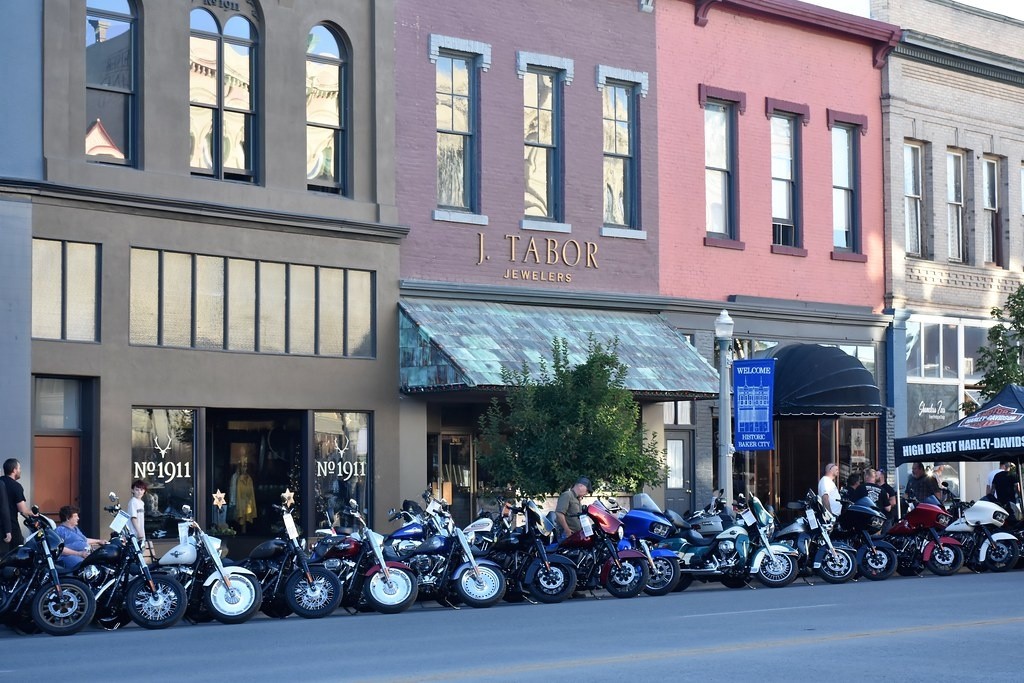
[879,468,884,473]
[829,464,836,470]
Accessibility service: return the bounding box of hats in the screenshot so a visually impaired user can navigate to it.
[576,477,595,493]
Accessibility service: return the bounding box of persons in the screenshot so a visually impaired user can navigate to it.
[556,478,594,546]
[932,462,947,504]
[126,480,146,554]
[905,462,941,503]
[840,469,896,540]
[986,461,1020,506]
[818,463,842,517]
[54,506,109,569]
[0,458,35,563]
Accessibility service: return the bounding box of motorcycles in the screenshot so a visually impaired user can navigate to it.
[135,504,264,624]
[0,505,97,637]
[15,493,188,631]
[311,481,1024,608]
[204,495,344,621]
[246,498,420,615]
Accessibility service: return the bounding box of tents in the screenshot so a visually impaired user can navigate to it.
[893,384,1024,525]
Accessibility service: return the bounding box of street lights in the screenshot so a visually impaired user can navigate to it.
[713,307,735,507]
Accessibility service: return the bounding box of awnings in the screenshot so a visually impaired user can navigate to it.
[749,341,883,417]
[398,298,735,400]
